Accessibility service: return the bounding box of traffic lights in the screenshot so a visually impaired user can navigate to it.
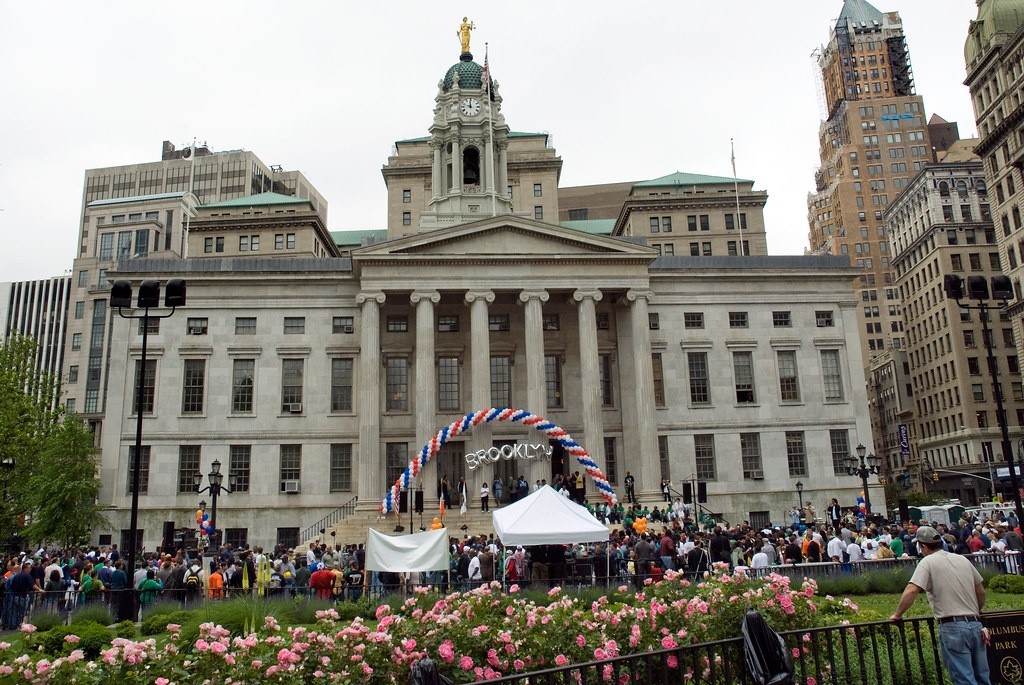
[936,473,939,481]
[933,473,936,481]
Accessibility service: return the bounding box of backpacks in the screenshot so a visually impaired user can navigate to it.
[186,567,202,589]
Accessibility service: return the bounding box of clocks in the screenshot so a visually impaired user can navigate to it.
[460,96,482,116]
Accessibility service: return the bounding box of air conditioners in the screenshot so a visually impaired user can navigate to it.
[285,481,300,491]
[914,388,917,391]
[872,399,875,402]
[817,319,826,326]
[344,326,354,332]
[751,470,763,479]
[868,401,870,404]
[290,403,302,411]
[193,328,201,333]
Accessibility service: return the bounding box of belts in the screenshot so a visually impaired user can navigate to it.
[936,614,982,625]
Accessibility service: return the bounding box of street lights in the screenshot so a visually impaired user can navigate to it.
[796,480,804,509]
[843,443,883,526]
[960,425,995,496]
[107,277,187,588]
[943,273,1024,541]
[193,459,238,557]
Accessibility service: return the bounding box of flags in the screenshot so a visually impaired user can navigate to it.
[394,484,400,520]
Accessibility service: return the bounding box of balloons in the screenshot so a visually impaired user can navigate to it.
[196,510,213,535]
[632,517,647,535]
[431,517,443,530]
[379,407,618,514]
[853,489,866,517]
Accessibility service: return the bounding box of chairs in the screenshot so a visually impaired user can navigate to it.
[547,555,593,590]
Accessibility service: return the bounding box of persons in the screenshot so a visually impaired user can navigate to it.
[439,470,717,521]
[0,521,1024,631]
[457,17,472,52]
[890,525,992,685]
[788,498,857,522]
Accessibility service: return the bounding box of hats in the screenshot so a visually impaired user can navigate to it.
[164,554,172,557]
[911,525,944,543]
[268,546,306,570]
[975,525,982,530]
[973,521,980,525]
[516,545,522,550]
[325,545,333,552]
[786,528,792,533]
[985,521,994,527]
[762,538,768,542]
[19,551,26,555]
[317,562,325,570]
[462,545,470,552]
[774,527,781,531]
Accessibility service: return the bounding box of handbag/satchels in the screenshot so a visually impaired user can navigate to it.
[163,574,178,589]
